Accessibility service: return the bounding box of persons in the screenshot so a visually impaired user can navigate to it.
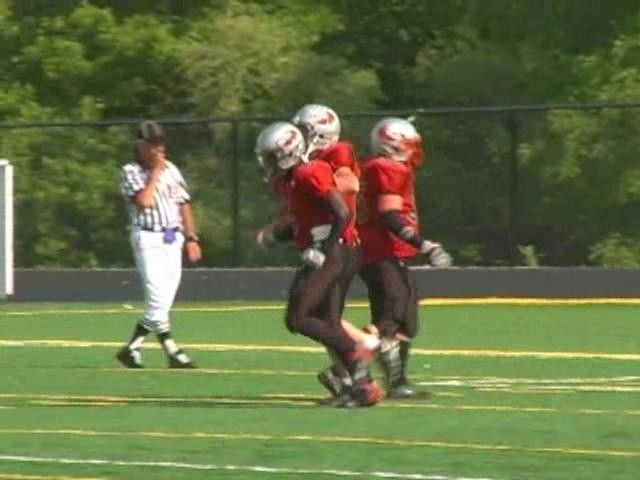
[116,121,202,368]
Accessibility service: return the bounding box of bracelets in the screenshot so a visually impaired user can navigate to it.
[185,234,199,242]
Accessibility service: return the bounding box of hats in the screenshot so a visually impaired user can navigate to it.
[136,120,166,144]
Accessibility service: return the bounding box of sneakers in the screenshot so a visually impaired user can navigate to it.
[318,364,345,398]
[386,382,433,401]
[375,336,403,388]
[167,348,199,370]
[316,379,388,410]
[117,345,145,370]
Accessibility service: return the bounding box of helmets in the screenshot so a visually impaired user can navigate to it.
[292,104,341,156]
[371,117,423,167]
[255,121,306,176]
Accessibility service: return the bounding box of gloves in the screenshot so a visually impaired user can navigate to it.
[303,248,326,270]
[421,240,443,260]
